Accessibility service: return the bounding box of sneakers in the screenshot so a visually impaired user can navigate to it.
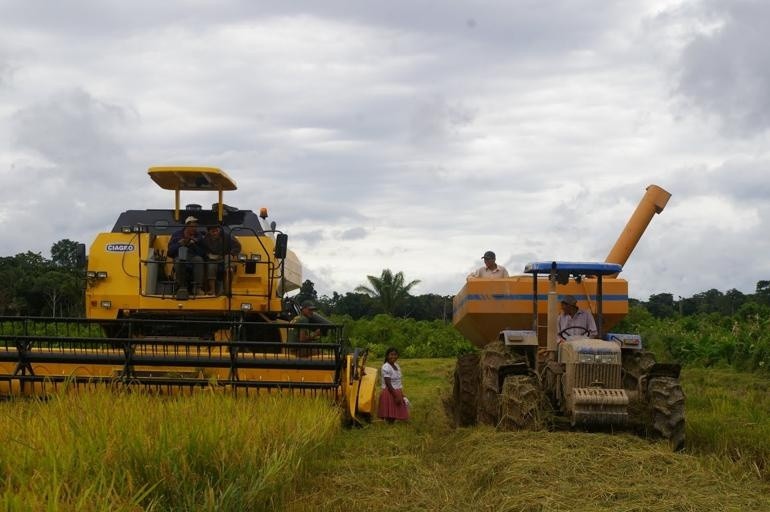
[177,288,216,295]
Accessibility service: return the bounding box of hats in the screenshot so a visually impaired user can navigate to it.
[561,295,577,306]
[302,300,316,309]
[185,216,198,224]
[481,251,495,259]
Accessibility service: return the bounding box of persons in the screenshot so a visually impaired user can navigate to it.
[556,295,599,345]
[377,348,409,424]
[166,216,241,296]
[287,299,332,357]
[465,251,510,279]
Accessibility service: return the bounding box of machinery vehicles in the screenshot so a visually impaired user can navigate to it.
[452,185,685,451]
[0,166,378,428]
